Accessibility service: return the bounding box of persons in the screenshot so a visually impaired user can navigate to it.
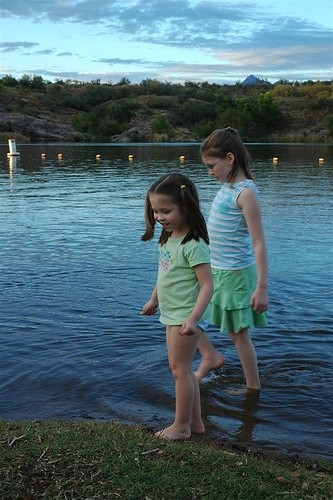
[191,127,273,396]
[138,173,217,441]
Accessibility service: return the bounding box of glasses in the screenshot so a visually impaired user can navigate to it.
[205,157,226,169]
